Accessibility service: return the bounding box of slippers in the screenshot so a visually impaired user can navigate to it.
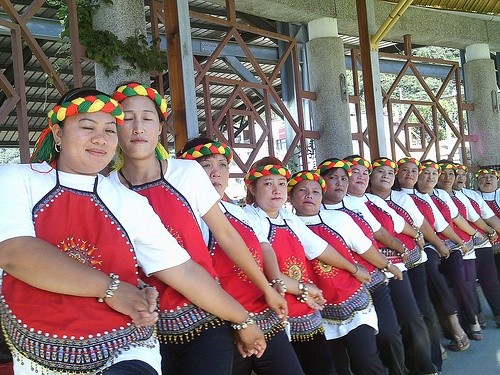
[440,345,447,360]
[452,329,470,351]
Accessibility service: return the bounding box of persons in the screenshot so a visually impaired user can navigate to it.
[0,81,500,375]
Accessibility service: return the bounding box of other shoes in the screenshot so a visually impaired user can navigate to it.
[449,341,458,350]
[479,319,486,328]
[496,322,500,325]
[469,332,483,340]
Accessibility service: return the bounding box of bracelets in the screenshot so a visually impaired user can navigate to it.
[413,228,423,242]
[230,318,253,330]
[471,229,478,239]
[456,240,464,249]
[488,229,496,237]
[138,284,152,290]
[396,244,408,257]
[379,260,391,273]
[98,272,120,303]
[268,278,287,294]
[351,265,359,276]
[296,281,309,303]
